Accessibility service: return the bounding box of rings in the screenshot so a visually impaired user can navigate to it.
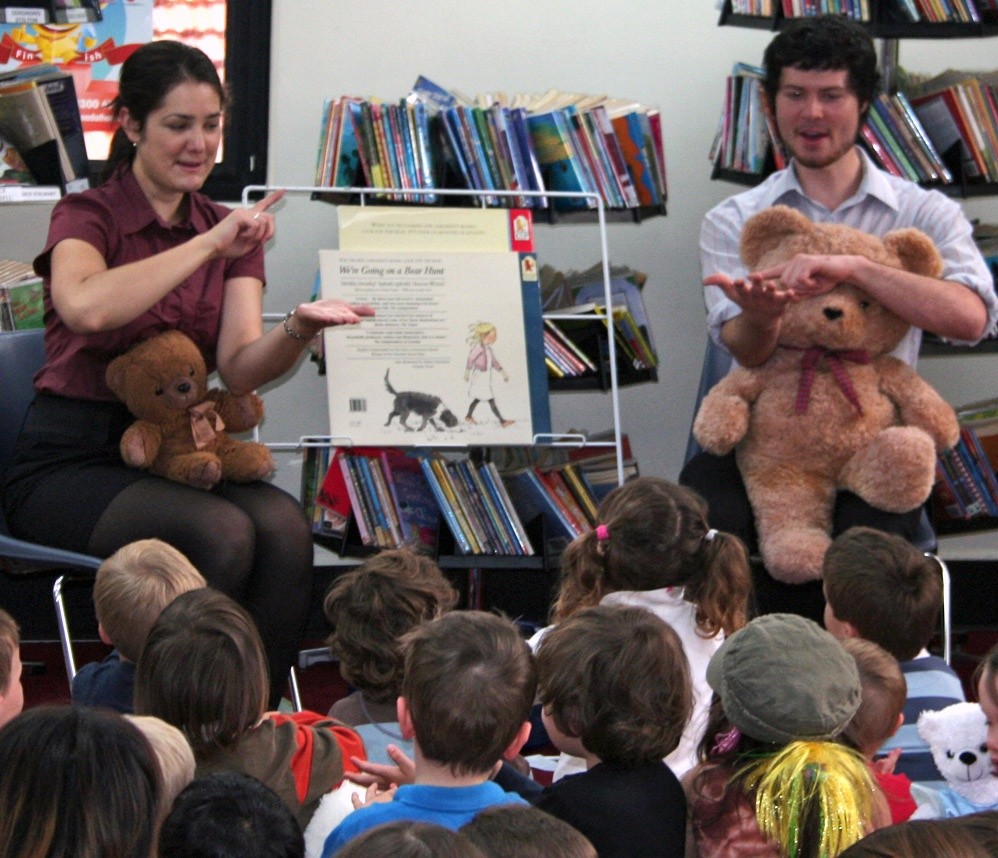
[253,212,260,219]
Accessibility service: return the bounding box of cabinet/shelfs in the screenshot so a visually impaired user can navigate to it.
[306,186,672,615]
[711,1,998,535]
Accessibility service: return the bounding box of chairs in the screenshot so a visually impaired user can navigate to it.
[0,331,104,696]
[684,336,952,666]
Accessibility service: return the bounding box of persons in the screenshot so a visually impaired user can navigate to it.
[0,40,377,711]
[0,474,998,858]
[699,12,998,631]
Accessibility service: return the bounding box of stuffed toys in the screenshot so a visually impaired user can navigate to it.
[917,700,998,818]
[692,205,960,586]
[105,331,275,492]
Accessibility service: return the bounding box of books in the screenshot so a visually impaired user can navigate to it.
[539,271,659,380]
[298,427,638,560]
[927,397,997,536]
[313,75,668,212]
[707,0,998,186]
[0,64,90,341]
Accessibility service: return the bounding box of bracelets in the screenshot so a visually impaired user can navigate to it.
[283,309,321,340]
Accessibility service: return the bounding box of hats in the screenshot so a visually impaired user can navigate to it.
[706,612,863,746]
